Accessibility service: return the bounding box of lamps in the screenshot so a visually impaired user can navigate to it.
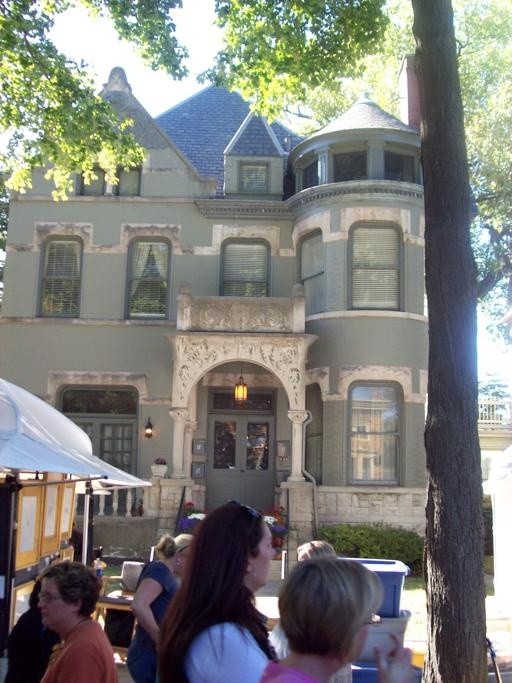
[235,363,248,403]
[145,417,152,437]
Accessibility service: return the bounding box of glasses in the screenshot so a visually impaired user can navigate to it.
[229,498,263,522]
[39,591,64,602]
[363,614,382,628]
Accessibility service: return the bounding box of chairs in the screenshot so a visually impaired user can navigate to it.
[93,562,145,661]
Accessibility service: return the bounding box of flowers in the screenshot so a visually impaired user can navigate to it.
[267,506,287,537]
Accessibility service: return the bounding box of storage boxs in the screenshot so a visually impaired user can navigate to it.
[335,557,411,683]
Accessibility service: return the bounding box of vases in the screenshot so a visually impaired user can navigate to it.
[273,536,281,547]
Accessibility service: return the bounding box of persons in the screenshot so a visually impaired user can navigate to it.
[3,564,61,683]
[69,513,83,563]
[261,554,416,683]
[160,501,278,683]
[126,531,194,682]
[35,561,121,683]
[269,539,337,659]
